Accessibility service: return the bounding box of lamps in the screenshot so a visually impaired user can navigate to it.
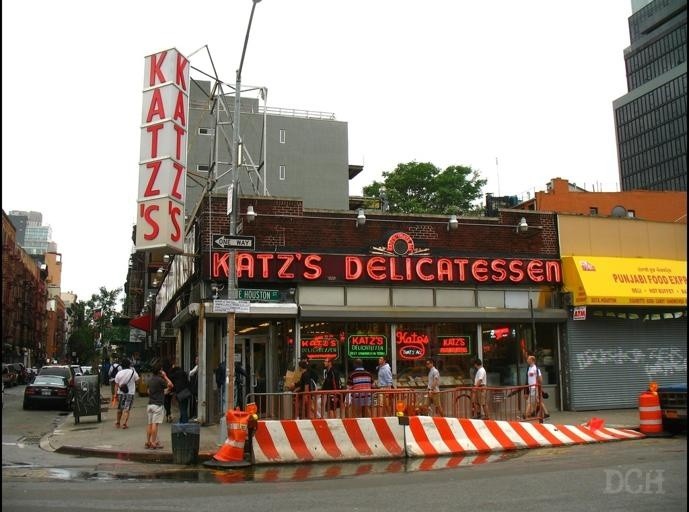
[138,252,176,324]
[243,204,547,236]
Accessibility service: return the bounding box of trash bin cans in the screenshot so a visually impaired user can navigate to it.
[171,423,200,465]
[74,375,101,424]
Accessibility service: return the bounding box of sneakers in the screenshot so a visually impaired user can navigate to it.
[544,413,550,419]
[115,423,128,429]
[145,441,164,449]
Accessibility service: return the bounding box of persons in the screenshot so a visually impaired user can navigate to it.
[289,354,548,420]
[108,358,251,450]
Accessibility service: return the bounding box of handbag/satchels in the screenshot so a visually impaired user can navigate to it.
[177,389,191,402]
[120,383,128,393]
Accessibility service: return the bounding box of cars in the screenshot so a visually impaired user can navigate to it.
[71,365,83,377]
[2,364,19,387]
[21,375,73,411]
[97,365,102,373]
[80,366,94,376]
[11,363,29,384]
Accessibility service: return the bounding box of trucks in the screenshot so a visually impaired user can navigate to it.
[648,382,687,420]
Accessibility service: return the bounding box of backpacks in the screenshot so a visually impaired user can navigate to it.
[111,364,120,378]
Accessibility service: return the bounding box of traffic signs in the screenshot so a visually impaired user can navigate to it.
[212,234,256,251]
[213,300,250,314]
[238,289,279,301]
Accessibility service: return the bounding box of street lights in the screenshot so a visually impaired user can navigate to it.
[218,0,263,446]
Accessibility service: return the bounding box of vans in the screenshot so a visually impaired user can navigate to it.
[401,364,464,380]
[36,365,75,386]
